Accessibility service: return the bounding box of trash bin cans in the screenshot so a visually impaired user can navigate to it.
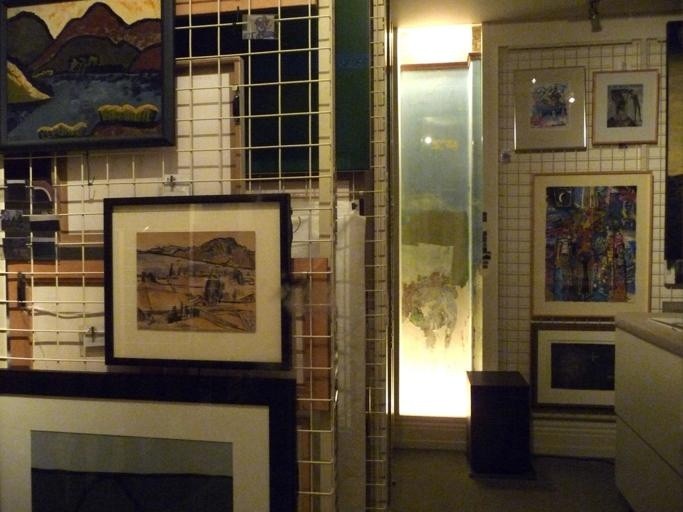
[467,370,531,478]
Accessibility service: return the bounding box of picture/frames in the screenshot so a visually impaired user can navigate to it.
[40,49,248,251]
[98,188,292,373]
[0,369,302,512]
[526,321,616,413]
[587,67,663,145]
[0,0,179,151]
[509,61,591,156]
[527,168,655,320]
